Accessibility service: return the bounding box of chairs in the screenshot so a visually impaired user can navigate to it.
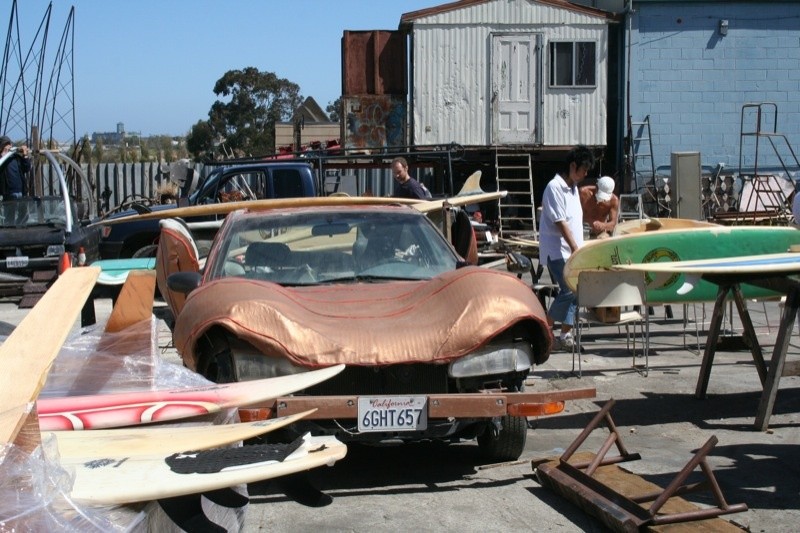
[572,271,649,378]
[244,242,291,278]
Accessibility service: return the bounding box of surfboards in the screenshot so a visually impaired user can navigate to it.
[612,249,800,275]
[86,170,509,260]
[34,361,348,508]
[561,217,800,304]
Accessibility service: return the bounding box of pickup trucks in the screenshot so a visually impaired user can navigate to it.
[65,161,509,261]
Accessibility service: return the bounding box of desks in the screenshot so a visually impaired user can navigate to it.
[614,251,800,432]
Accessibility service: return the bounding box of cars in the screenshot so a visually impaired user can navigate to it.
[0,193,101,275]
[156,206,596,464]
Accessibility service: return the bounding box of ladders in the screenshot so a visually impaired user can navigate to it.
[630,114,661,219]
[495,151,538,246]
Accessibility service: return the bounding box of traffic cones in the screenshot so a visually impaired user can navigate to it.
[78,248,89,267]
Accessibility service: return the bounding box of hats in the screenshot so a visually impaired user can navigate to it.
[597,176,615,200]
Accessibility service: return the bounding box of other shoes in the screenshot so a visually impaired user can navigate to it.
[558,335,584,351]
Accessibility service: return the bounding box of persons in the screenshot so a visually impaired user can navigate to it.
[536,145,595,353]
[578,176,620,237]
[387,156,433,200]
[0,135,32,223]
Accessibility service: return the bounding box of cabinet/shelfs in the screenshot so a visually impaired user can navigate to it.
[671,151,702,220]
